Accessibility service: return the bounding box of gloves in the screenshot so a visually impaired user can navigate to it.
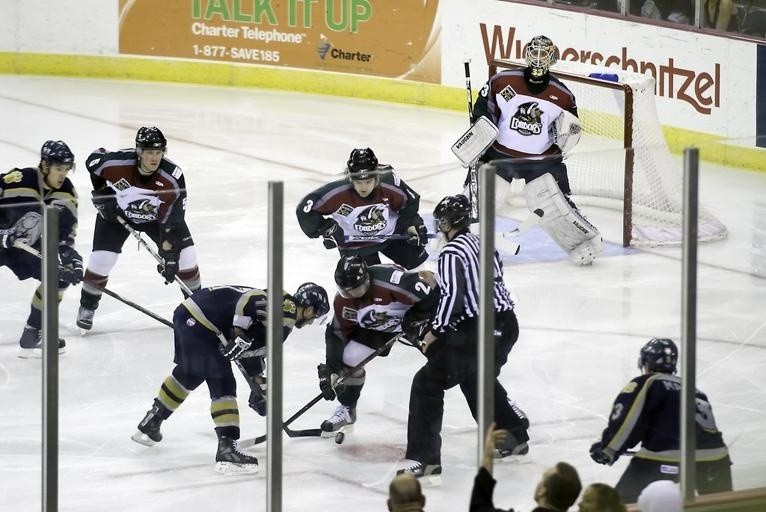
[589,442,620,466]
[156,250,180,284]
[1,228,29,251]
[59,249,84,286]
[317,363,344,401]
[318,217,345,250]
[247,377,267,417]
[90,186,119,224]
[217,333,255,361]
[404,215,428,248]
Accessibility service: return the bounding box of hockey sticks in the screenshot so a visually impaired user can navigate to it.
[461,58,522,259]
[237,331,406,450]
[216,330,322,438]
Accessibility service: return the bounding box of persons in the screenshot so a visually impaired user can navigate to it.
[468,421,583,512]
[387,473,426,512]
[577,483,625,511]
[130,282,330,475]
[76,125,202,337]
[588,337,734,504]
[461,34,604,267]
[0,140,84,360]
[395,194,531,488]
[319,254,438,438]
[295,146,430,271]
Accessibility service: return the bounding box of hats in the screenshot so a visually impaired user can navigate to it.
[636,479,684,512]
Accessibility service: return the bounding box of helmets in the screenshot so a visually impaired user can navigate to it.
[525,35,560,69]
[135,125,167,151]
[293,281,330,316]
[40,139,74,165]
[637,337,679,372]
[346,146,380,180]
[432,194,472,220]
[335,253,369,288]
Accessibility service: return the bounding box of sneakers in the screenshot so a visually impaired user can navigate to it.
[510,404,529,429]
[396,462,442,478]
[320,406,358,432]
[137,409,164,443]
[215,436,260,465]
[19,326,66,349]
[493,441,530,459]
[76,306,95,331]
